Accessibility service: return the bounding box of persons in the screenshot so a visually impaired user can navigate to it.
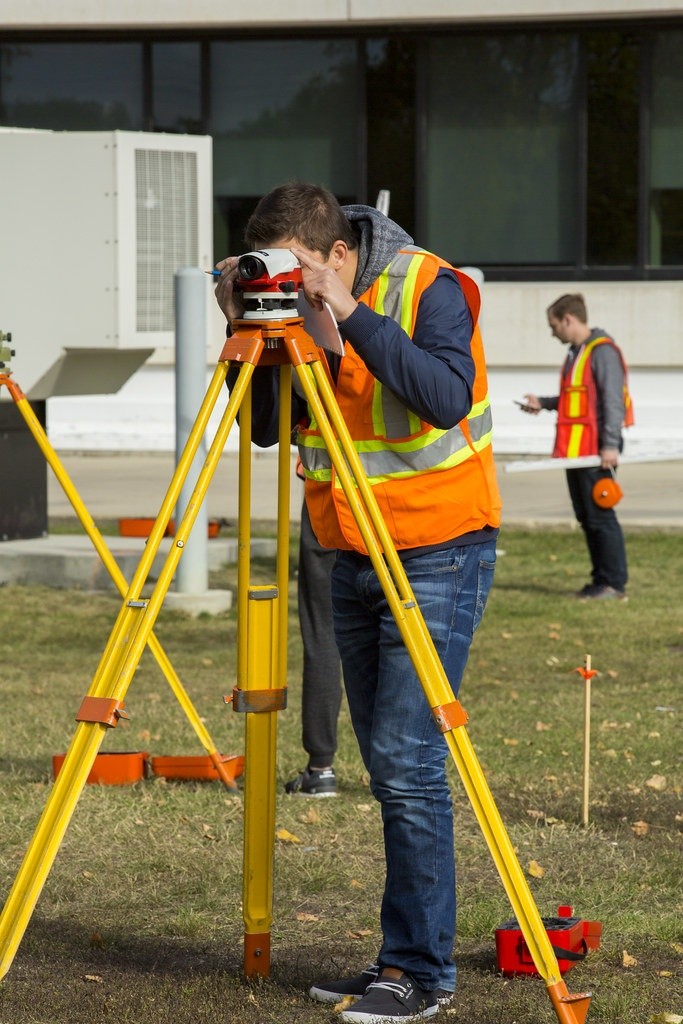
[215,183,502,1024]
[520,294,636,602]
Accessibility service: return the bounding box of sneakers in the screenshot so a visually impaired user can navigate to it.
[309,961,452,1024]
[285,764,339,797]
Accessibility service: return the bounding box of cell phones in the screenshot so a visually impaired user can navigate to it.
[514,400,539,411]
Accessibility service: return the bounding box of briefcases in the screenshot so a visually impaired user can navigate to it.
[495,916,588,973]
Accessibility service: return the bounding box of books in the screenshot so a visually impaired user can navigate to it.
[295,288,345,356]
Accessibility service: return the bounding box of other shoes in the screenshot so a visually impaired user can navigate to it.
[573,583,628,604]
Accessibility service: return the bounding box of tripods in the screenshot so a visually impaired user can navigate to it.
[0,319,589,1024]
